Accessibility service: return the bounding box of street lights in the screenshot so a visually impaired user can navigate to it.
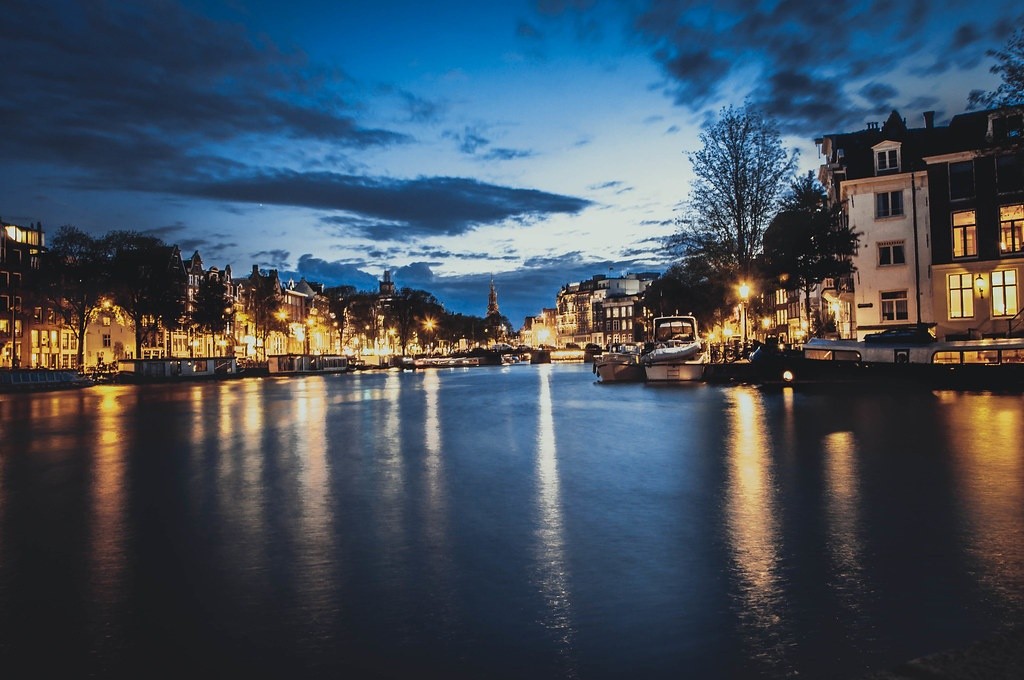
[426,320,433,356]
[739,281,749,359]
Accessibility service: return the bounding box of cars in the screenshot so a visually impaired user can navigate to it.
[864,324,934,343]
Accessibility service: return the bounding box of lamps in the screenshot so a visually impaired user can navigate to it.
[976,273,985,298]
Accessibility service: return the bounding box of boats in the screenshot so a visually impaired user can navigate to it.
[0,367,95,393]
[348,363,357,372]
[400,358,480,369]
[768,340,1024,391]
[263,354,349,376]
[113,358,246,384]
[593,315,709,381]
